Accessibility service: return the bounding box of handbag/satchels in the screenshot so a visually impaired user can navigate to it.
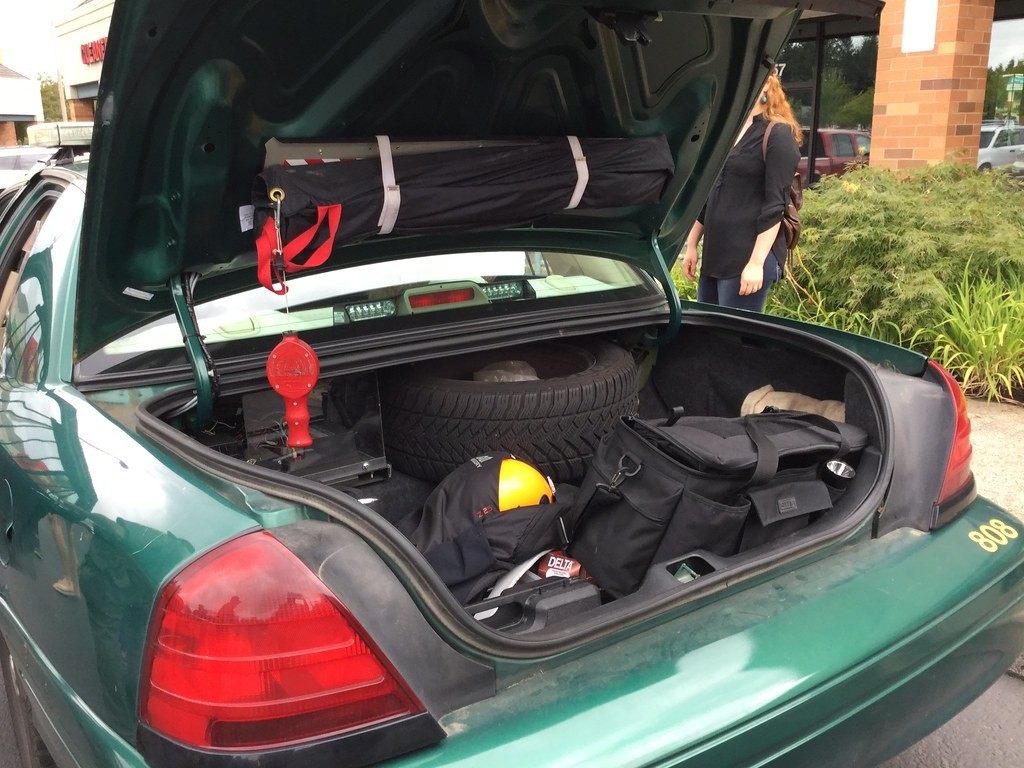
[555,406,870,599]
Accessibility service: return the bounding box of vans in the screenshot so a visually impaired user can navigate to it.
[976,125,1024,172]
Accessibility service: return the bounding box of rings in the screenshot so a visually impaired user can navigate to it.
[752,287,757,292]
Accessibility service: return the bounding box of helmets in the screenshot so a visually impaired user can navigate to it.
[496,452,557,511]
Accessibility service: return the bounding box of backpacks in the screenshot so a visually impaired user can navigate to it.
[762,120,803,251]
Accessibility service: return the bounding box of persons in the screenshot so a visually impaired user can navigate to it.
[683,66,803,311]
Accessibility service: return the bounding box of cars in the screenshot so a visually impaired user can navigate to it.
[1,0,1024,767]
[0,146,91,190]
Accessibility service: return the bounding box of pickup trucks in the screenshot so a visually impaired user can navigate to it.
[797,128,871,189]
